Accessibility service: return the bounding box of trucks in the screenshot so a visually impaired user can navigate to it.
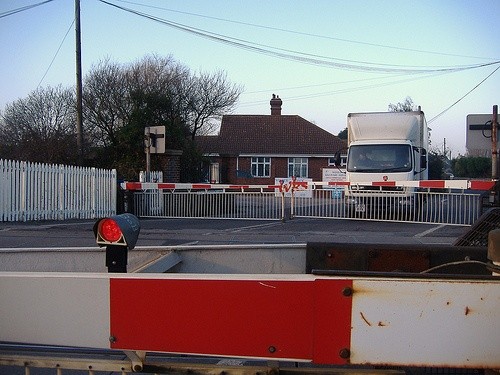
[332,111,429,215]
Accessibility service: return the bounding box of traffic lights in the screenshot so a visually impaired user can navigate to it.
[92,213,141,252]
